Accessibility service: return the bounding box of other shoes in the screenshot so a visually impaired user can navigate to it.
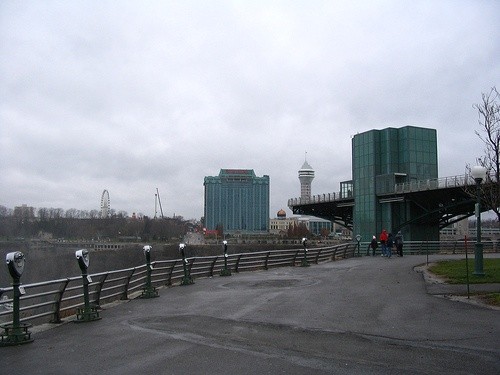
[384,254,387,256]
[381,254,384,256]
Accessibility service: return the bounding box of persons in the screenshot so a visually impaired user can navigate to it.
[370,229,404,258]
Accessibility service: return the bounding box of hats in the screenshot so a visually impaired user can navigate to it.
[373,235,376,239]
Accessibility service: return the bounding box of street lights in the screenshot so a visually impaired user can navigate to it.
[471,165,486,283]
